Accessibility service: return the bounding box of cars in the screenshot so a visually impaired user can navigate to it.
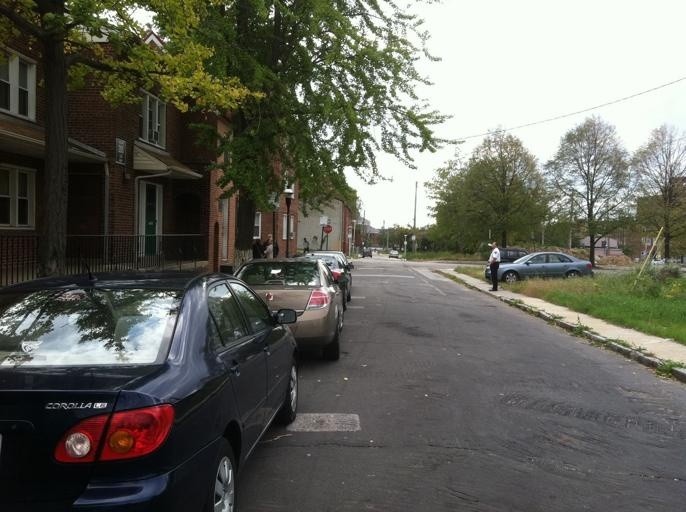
[362,248,372,258]
[389,249,399,258]
[484,251,594,284]
[653,258,666,265]
[0,267,304,512]
[230,249,355,362]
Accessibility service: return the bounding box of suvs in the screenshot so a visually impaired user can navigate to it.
[498,248,530,263]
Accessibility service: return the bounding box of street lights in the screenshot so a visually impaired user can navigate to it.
[282,186,294,258]
[404,235,408,256]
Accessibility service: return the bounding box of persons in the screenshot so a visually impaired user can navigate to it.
[303,237,309,253]
[487,241,502,291]
[252,234,281,262]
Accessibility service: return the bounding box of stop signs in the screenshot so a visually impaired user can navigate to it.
[323,225,332,233]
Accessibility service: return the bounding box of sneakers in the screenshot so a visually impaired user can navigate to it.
[489,289,497,291]
